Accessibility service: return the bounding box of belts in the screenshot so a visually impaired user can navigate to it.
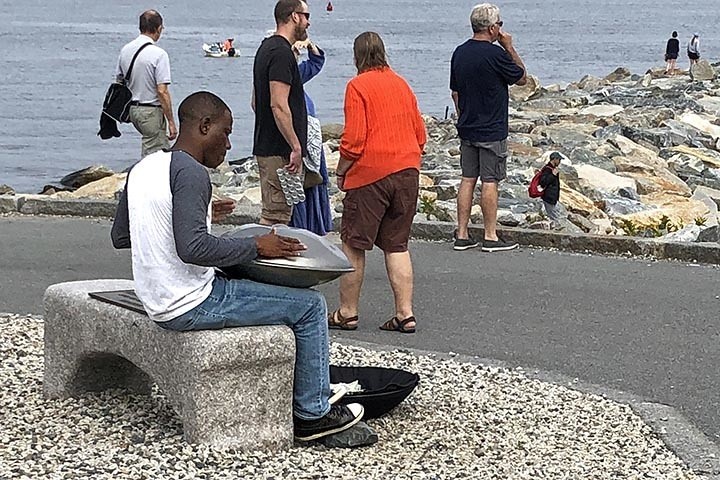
[131,101,162,108]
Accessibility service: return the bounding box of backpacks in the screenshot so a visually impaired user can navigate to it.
[529,166,553,198]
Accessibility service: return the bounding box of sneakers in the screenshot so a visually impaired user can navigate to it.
[293,402,365,441]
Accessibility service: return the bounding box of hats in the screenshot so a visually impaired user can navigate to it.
[694,32,699,37]
[550,151,565,160]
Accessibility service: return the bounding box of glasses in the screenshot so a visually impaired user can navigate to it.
[288,11,310,21]
[495,21,504,26]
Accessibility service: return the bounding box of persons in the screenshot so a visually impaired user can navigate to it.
[110,92,365,440]
[664,31,680,75]
[542,152,565,218]
[687,33,702,69]
[110,9,177,250]
[450,3,527,252]
[217,0,333,238]
[328,32,430,331]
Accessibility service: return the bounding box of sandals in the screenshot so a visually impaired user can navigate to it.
[379,316,416,333]
[327,308,358,329]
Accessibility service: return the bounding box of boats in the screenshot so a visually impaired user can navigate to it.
[202,41,240,57]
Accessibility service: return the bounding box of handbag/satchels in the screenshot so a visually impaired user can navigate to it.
[103,83,132,123]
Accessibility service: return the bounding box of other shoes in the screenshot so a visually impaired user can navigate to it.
[664,71,667,74]
[454,238,479,249]
[483,238,519,252]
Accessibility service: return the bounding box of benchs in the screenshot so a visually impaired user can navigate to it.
[44,279,297,451]
